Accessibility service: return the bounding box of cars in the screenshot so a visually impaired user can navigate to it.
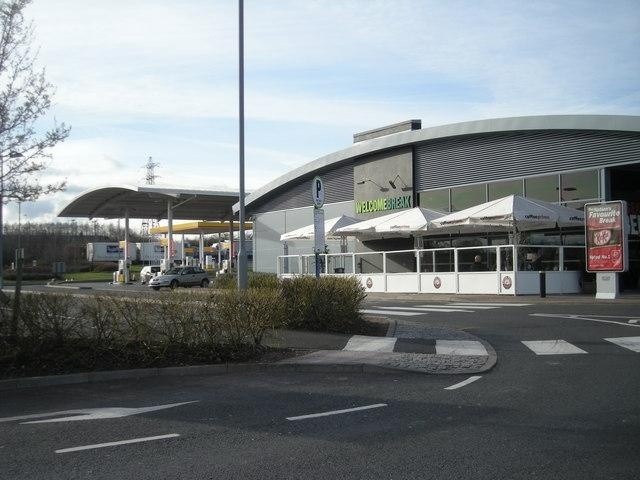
[140,265,211,290]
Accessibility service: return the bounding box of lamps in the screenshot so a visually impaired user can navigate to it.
[389,175,399,189]
[357,180,371,185]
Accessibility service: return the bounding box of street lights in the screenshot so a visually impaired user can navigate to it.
[0,153,24,289]
